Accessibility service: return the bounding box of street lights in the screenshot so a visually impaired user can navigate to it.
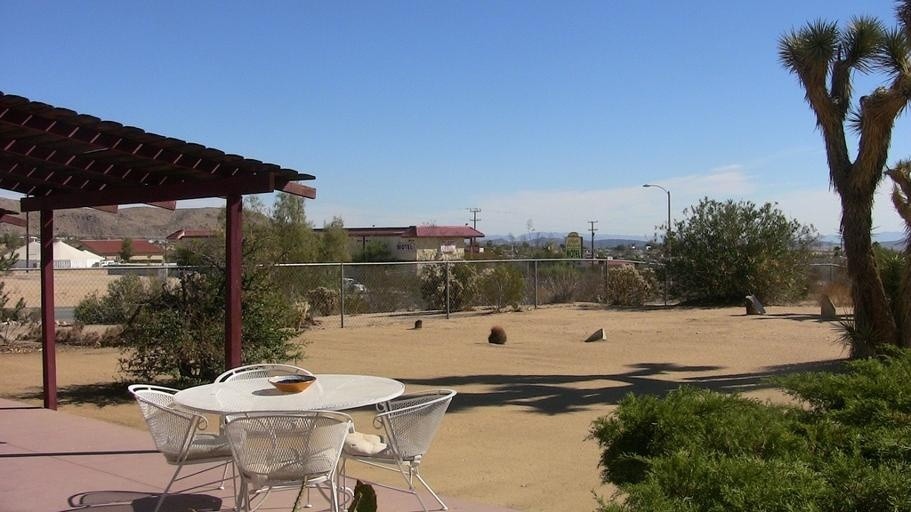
[643,184,673,288]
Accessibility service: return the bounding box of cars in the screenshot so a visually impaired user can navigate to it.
[343,278,367,293]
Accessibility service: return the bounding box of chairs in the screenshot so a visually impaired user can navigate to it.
[213,363,325,508]
[127,384,251,512]
[332,387,459,512]
[220,409,355,512]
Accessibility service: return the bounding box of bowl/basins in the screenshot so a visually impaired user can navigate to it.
[269,374,318,393]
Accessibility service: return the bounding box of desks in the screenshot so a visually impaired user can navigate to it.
[173,373,406,512]
